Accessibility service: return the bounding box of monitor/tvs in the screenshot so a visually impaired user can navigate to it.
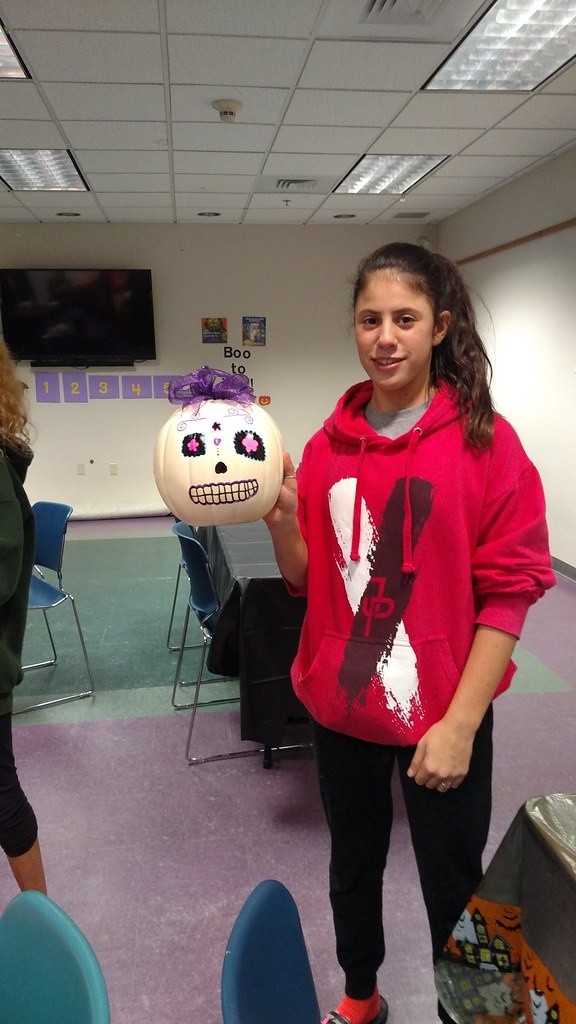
[0,268,157,368]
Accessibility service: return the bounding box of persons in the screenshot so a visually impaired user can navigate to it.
[265,242,559,1024]
[0,334,46,897]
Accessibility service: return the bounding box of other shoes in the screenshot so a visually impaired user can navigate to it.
[320,994,388,1024]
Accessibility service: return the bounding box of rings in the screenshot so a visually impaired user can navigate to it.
[284,475,296,479]
[440,782,450,789]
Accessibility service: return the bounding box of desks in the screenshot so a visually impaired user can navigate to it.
[434,793,576,1024]
[196,519,311,769]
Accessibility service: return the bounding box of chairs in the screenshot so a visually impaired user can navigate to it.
[166,517,315,763]
[11,501,94,715]
[0,890,112,1024]
[221,880,322,1024]
